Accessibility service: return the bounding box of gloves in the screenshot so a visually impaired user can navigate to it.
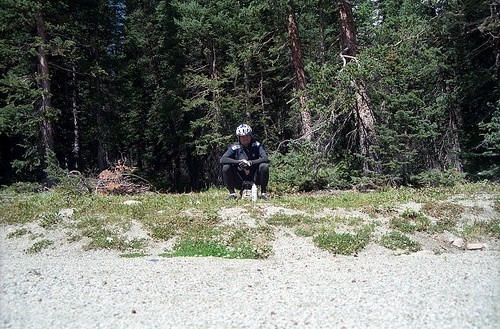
[239,159,250,170]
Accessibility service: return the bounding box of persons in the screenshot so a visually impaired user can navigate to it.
[220,124,270,200]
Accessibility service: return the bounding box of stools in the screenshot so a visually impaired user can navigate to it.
[241,181,261,201]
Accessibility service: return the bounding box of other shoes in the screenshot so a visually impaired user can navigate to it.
[260,193,270,201]
[226,193,237,201]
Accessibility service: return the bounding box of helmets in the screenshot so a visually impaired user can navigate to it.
[236,123,252,136]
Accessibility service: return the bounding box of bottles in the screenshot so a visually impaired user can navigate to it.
[252,184,257,202]
